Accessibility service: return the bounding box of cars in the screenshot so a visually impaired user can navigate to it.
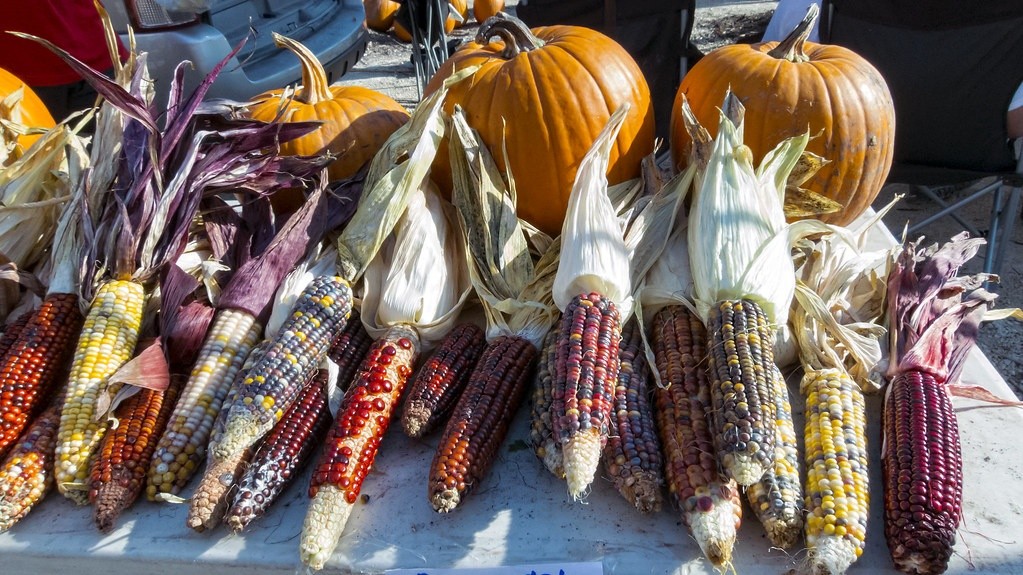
[99,1,374,117]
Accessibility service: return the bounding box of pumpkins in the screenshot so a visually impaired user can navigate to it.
[416,12,655,238]
[668,2,896,225]
[360,0,506,42]
[237,31,410,217]
[0,67,58,167]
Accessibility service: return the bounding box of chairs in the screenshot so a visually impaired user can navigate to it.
[518,1,705,139]
[820,0,1023,310]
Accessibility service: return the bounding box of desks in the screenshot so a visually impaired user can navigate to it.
[1,207,1023,575]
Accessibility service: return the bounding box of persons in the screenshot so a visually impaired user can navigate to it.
[0,0,131,155]
[758,0,823,44]
[907,82,1023,201]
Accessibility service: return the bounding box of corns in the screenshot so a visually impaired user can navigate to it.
[0,278,964,574]
